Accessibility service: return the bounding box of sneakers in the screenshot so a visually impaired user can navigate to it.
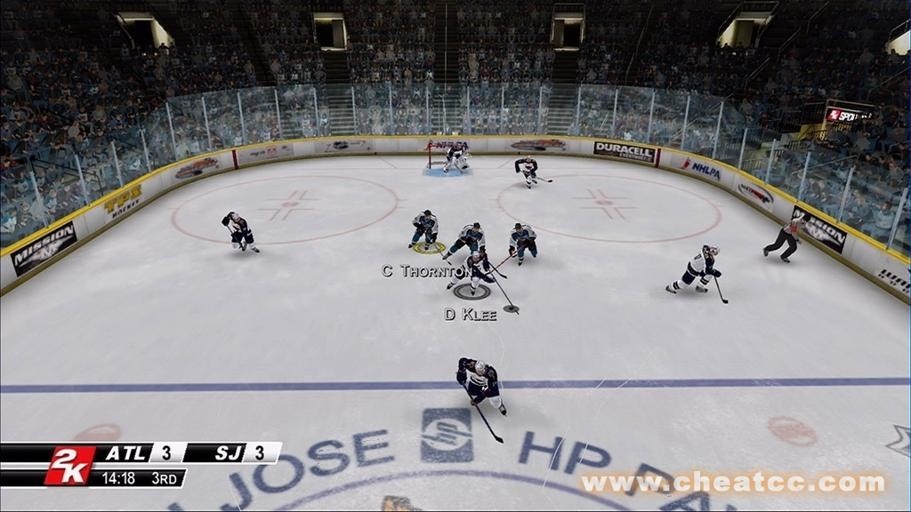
[695,287,709,292]
[764,247,769,256]
[407,241,537,296]
[498,401,507,415]
[666,286,677,295]
[780,255,791,263]
[242,243,248,252]
[251,247,259,253]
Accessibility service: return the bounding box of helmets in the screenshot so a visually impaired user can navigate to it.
[711,243,720,256]
[423,209,523,261]
[230,213,240,220]
[474,360,486,375]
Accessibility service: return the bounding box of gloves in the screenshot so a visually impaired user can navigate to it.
[456,371,467,385]
[222,216,230,226]
[714,269,722,278]
[471,399,481,405]
[231,232,242,244]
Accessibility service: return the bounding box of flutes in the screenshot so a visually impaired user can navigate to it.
[463,383,504,444]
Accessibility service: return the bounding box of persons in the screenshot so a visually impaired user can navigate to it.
[446,250,495,295]
[666,245,721,292]
[456,357,507,416]
